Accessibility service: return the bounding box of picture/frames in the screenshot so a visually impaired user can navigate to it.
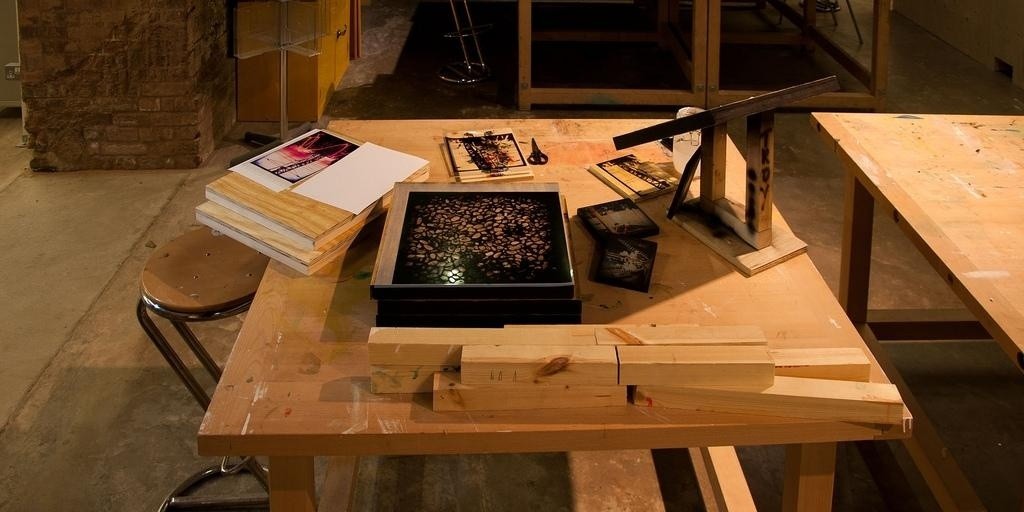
[369,182,581,327]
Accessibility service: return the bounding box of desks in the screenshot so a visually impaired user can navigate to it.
[516,0,708,112]
[196,124,913,511]
[813,112,1024,512]
[708,1,891,111]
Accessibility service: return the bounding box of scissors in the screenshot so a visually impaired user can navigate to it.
[527,138,548,165]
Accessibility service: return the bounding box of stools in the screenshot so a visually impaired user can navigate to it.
[436,0,489,85]
[132,227,271,512]
[800,1,864,49]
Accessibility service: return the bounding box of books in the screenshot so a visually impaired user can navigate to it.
[588,154,679,203]
[443,128,534,184]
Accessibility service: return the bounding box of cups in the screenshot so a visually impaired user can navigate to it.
[671,105,709,178]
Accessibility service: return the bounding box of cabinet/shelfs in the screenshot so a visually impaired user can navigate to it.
[232,0,352,126]
